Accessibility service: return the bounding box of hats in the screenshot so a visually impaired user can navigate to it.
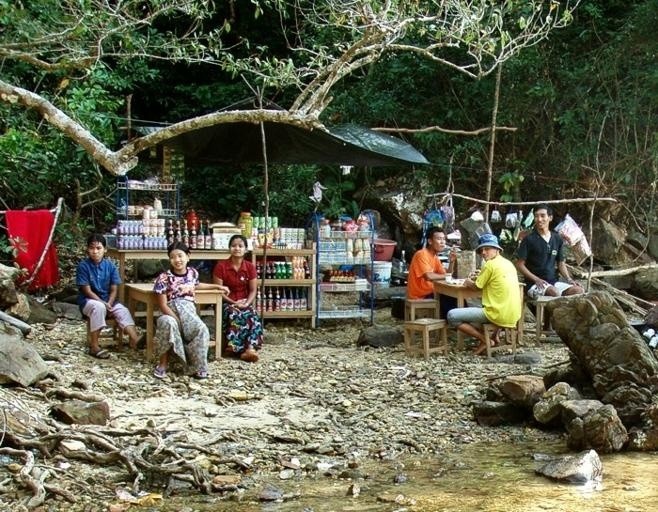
[476,234,504,253]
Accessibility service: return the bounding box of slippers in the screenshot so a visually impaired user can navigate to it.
[154,365,166,378]
[196,370,207,377]
[91,349,110,359]
[242,351,258,361]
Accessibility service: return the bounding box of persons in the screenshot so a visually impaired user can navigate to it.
[212,235,265,362]
[153,241,231,379]
[407,226,458,300]
[514,203,585,299]
[447,233,523,356]
[76,234,146,360]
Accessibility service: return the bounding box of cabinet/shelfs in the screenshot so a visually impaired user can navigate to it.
[250,240,318,330]
[314,210,375,329]
[115,172,182,222]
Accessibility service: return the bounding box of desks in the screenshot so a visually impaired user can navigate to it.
[123,282,228,363]
[108,242,249,351]
[433,279,527,351]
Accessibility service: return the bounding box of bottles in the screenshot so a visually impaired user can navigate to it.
[124,221,128,234]
[257,287,261,312]
[134,235,138,250]
[399,251,407,276]
[153,236,158,249]
[124,235,129,250]
[273,217,277,228]
[182,220,189,249]
[286,286,294,312]
[159,236,163,250]
[138,235,143,250]
[303,260,308,270]
[196,220,204,250]
[149,235,154,250]
[175,221,182,243]
[129,235,133,250]
[117,235,124,250]
[262,294,266,311]
[294,287,301,311]
[280,286,288,311]
[306,266,310,279]
[144,235,149,249]
[167,219,174,248]
[267,286,273,311]
[267,217,271,228]
[324,220,330,240]
[287,262,294,279]
[204,220,212,250]
[161,235,168,249]
[301,286,307,311]
[238,213,252,239]
[260,216,265,229]
[256,261,276,280]
[275,262,281,279]
[117,220,123,234]
[128,220,134,235]
[274,287,280,311]
[139,221,143,234]
[189,220,197,249]
[254,217,259,228]
[134,221,138,234]
[281,262,287,279]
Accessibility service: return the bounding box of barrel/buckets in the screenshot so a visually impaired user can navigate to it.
[373,260,393,288]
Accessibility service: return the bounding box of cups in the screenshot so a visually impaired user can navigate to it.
[150,227,157,237]
[142,219,150,227]
[143,210,150,218]
[143,227,150,237]
[157,219,165,227]
[150,219,157,227]
[151,210,158,218]
[157,227,165,237]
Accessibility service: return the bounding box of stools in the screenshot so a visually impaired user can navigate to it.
[86,317,123,352]
[484,323,524,357]
[406,318,448,359]
[535,295,565,342]
[405,298,438,320]
